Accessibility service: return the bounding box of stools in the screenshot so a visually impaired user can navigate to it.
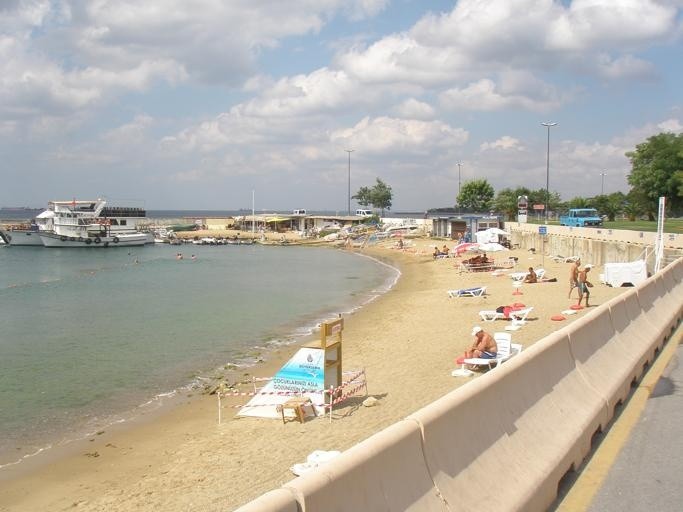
[280,397,317,425]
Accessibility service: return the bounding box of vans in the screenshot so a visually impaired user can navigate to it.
[355,210,372,218]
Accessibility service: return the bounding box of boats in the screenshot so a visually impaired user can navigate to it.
[32,199,147,248]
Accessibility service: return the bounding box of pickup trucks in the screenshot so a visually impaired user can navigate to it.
[559,208,602,227]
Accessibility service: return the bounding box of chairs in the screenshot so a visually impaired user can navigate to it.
[461,332,522,371]
[478,306,534,322]
[447,286,489,297]
[462,261,495,272]
[511,268,545,281]
[301,317,344,414]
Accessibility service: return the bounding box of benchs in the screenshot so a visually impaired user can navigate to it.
[434,254,449,260]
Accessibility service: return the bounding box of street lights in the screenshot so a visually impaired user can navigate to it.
[455,159,463,218]
[541,122,557,224]
[343,145,355,216]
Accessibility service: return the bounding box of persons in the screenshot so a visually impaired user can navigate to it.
[577,266,595,308]
[432,231,489,272]
[396,239,402,248]
[521,265,537,284]
[175,254,180,260]
[465,326,499,370]
[179,254,182,259]
[191,254,196,260]
[567,260,582,299]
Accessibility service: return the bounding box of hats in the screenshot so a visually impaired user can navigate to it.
[585,264,592,269]
[472,327,482,336]
